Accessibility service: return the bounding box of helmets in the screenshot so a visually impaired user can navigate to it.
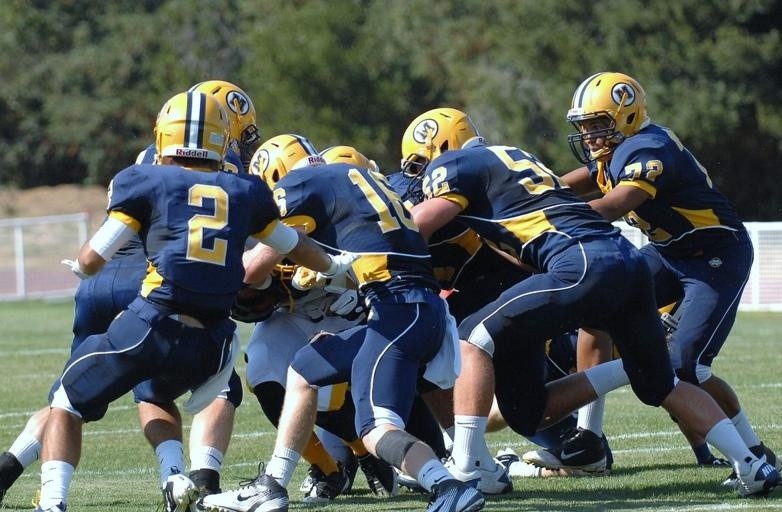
[322,143,382,172]
[189,81,260,161]
[564,70,649,163]
[249,132,321,189]
[153,92,231,167]
[401,105,484,197]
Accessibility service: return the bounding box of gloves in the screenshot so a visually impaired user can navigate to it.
[323,284,366,322]
[316,252,360,284]
[62,258,90,281]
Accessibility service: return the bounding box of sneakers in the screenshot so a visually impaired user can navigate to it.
[398,458,515,498]
[721,442,777,488]
[300,462,321,491]
[189,469,220,510]
[154,467,198,512]
[197,463,291,512]
[698,451,734,469]
[357,453,400,499]
[602,435,613,477]
[424,480,485,512]
[523,428,608,473]
[24,498,64,512]
[300,465,351,505]
[731,460,782,496]
[496,448,516,467]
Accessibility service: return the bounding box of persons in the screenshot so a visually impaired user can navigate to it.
[264,172,612,478]
[28,89,360,512]
[523,71,782,487]
[1,80,291,512]
[398,107,781,500]
[192,134,485,512]
